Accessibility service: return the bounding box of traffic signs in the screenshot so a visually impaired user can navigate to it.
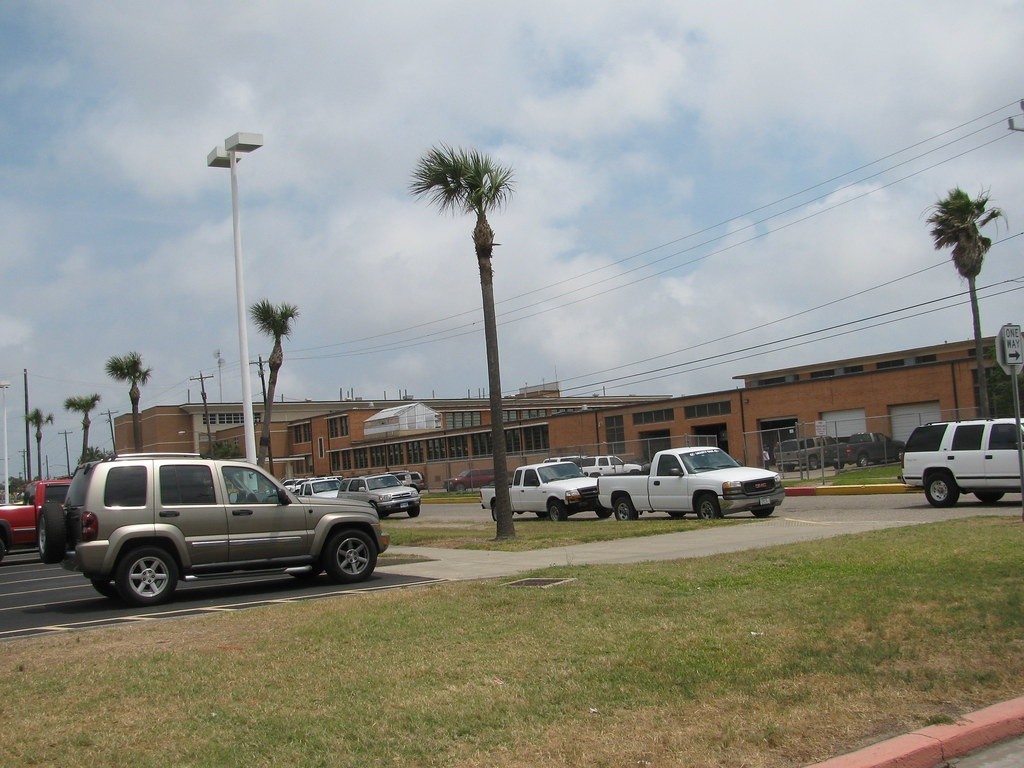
[1003,324,1024,366]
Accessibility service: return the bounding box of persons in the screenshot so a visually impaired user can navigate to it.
[762,447,770,470]
[773,442,782,471]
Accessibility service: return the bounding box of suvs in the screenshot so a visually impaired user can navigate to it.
[36,451,391,609]
[386,470,426,493]
[442,467,496,493]
[543,455,643,479]
[899,417,1024,508]
[336,472,421,519]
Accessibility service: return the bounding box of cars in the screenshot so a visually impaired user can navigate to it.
[264,475,346,500]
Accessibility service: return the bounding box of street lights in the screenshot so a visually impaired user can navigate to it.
[207,131,265,493]
[0,381,11,505]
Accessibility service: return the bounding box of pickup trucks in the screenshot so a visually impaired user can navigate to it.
[596,445,785,521]
[478,461,614,522]
[0,479,72,564]
[831,431,906,469]
[774,434,840,471]
